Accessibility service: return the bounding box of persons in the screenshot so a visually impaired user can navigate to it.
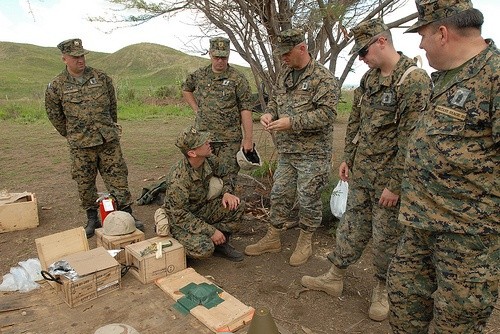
[44,38,145,238]
[163,125,247,262]
[244,27,341,267]
[300,17,433,323]
[181,37,255,195]
[386,0,500,334]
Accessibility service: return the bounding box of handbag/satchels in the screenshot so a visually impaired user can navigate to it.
[329,178,348,219]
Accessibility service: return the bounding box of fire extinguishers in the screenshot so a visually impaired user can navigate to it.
[95,192,116,226]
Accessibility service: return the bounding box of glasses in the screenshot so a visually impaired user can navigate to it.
[357,38,387,56]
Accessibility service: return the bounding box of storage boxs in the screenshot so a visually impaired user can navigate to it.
[93,222,146,255]
[33,225,124,311]
[0,191,41,234]
[124,235,186,286]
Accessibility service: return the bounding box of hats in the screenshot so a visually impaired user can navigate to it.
[272,27,305,55]
[236,145,261,170]
[209,37,230,57]
[102,211,136,236]
[347,18,389,56]
[174,126,211,151]
[57,39,90,57]
[402,0,474,34]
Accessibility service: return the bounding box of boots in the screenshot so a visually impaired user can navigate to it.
[213,231,242,258]
[117,204,144,230]
[301,263,347,297]
[368,278,389,322]
[289,228,313,266]
[84,208,102,238]
[244,225,282,255]
